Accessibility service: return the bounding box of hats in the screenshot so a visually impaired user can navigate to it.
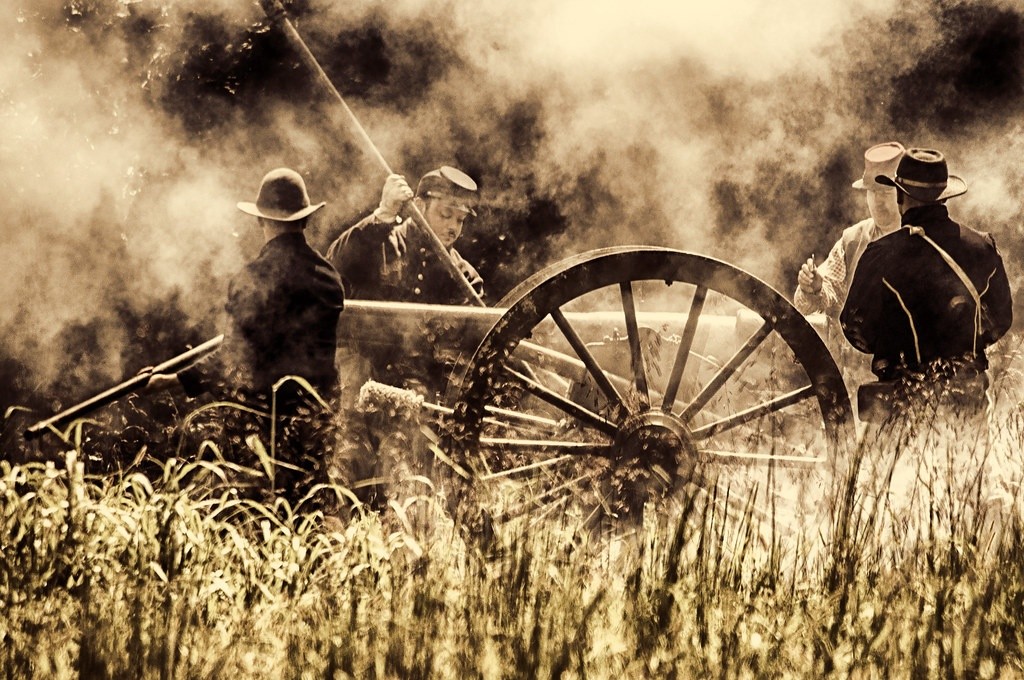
[851,142,907,190]
[875,148,968,201]
[416,166,483,205]
[236,168,326,221]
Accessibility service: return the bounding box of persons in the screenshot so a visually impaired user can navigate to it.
[133,166,346,515]
[795,140,1015,447]
[326,165,489,508]
